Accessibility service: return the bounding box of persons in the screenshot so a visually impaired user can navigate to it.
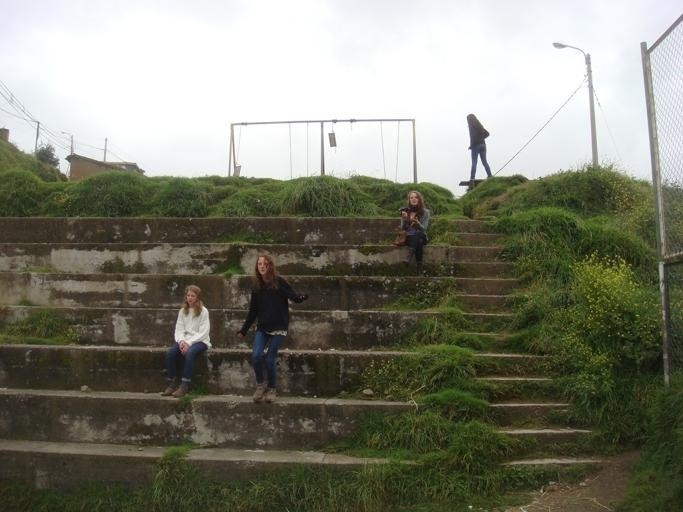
[236,254,307,403]
[467,114,493,181]
[164,284,213,396]
[401,189,432,275]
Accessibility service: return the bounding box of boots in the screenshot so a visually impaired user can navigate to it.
[417,263,424,277]
[402,248,415,267]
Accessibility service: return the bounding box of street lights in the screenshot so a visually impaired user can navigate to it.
[551,40,601,172]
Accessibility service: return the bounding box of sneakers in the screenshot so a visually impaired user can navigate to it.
[161,387,187,397]
[253,383,276,402]
